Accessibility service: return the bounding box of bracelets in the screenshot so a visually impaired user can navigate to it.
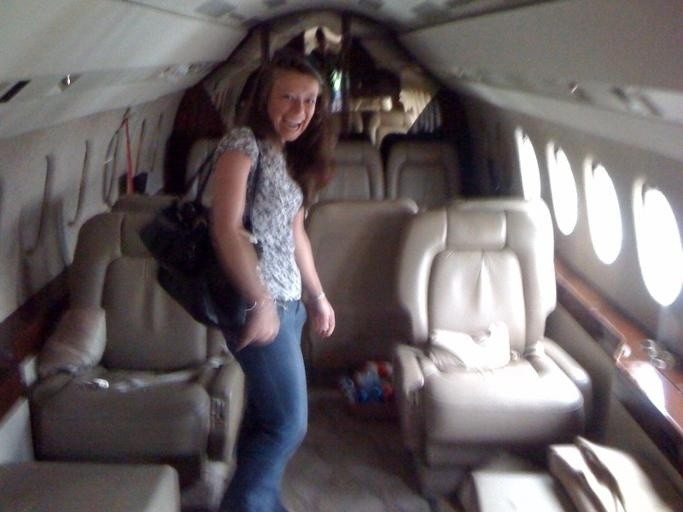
[243,297,275,312]
[304,292,325,305]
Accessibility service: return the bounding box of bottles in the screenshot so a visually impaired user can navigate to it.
[336,362,394,407]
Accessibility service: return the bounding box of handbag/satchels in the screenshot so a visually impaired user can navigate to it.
[138,125,265,330]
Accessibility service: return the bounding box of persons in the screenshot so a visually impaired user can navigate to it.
[209,50,339,511]
[309,28,341,109]
